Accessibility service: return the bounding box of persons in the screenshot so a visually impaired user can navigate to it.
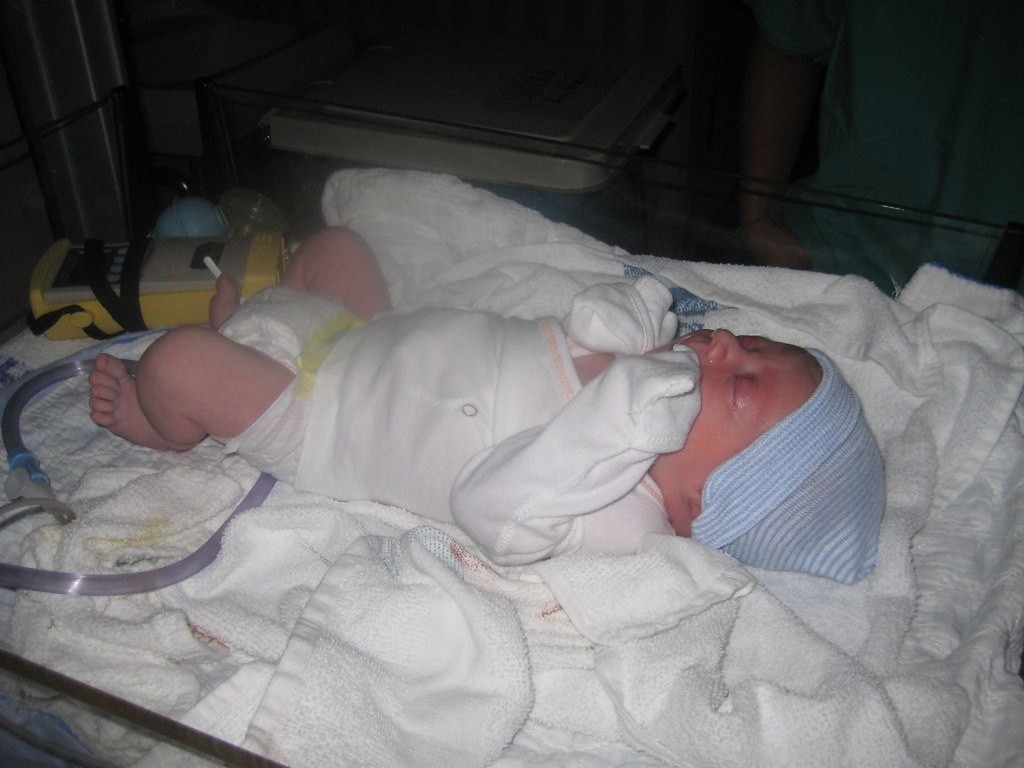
[741,0,1024,292]
[88,225,885,583]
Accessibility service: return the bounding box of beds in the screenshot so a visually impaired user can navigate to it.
[0,58,1024,768]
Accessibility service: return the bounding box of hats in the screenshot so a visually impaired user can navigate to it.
[690,348,886,585]
